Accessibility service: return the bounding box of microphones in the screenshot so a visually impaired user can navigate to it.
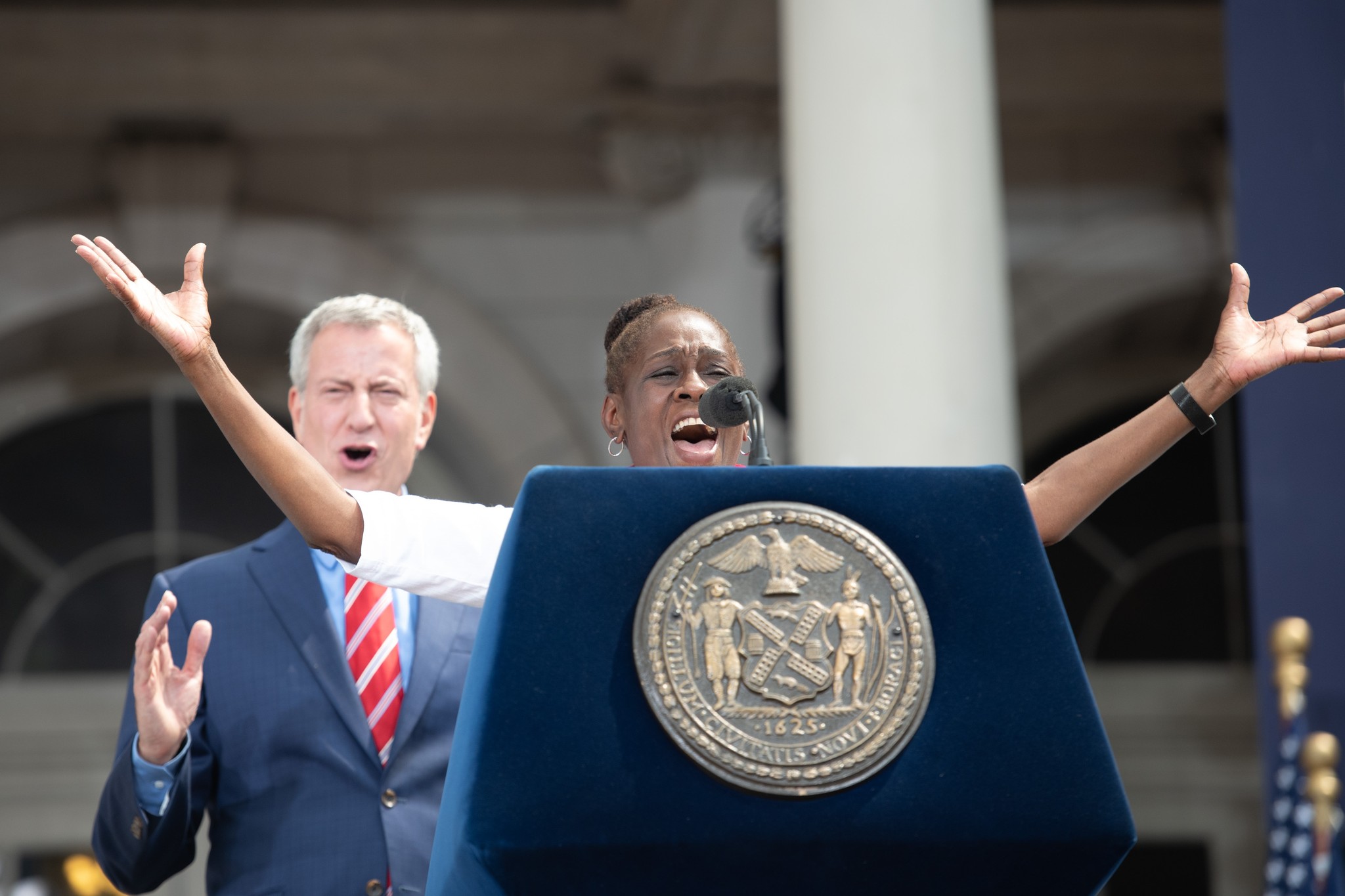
[697,377,771,467]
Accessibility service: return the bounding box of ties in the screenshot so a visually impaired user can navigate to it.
[342,572,406,896]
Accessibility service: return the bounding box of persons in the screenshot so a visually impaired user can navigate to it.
[72,233,1345,611]
[91,292,485,896]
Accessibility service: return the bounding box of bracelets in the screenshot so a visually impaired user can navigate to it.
[1169,382,1217,435]
